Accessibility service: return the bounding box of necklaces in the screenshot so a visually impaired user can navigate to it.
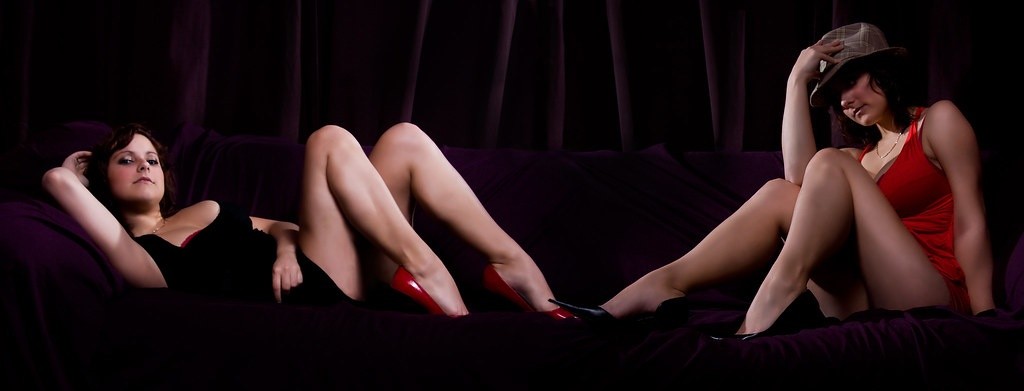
[150,218,167,235]
[876,131,901,158]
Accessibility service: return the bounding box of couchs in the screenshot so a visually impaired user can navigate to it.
[0,121,1024,391]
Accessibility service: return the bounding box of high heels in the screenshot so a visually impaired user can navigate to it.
[710,287,816,340]
[483,262,579,318]
[389,267,469,318]
[548,294,689,325]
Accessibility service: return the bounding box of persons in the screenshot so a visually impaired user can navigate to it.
[40,120,581,321]
[549,23,999,341]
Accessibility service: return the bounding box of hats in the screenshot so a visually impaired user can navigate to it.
[809,22,912,109]
